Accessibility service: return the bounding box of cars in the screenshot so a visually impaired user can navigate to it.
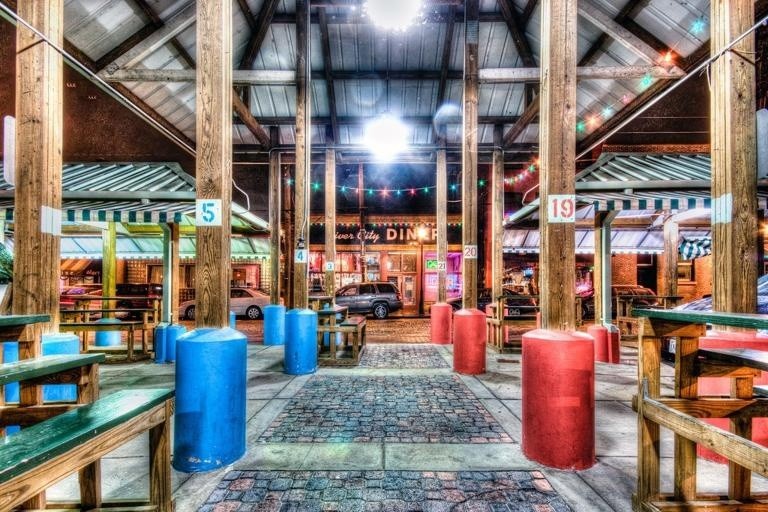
[661,274,768,363]
[574,285,657,320]
[427,287,537,321]
[60,282,285,320]
[321,281,404,319]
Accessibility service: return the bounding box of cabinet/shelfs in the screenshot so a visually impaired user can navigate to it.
[0,313,176,512]
[308,295,366,366]
[491,295,582,354]
[58,297,163,364]
[631,309,768,512]
[616,295,683,341]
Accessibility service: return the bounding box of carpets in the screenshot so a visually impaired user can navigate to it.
[318,343,451,368]
[195,470,575,512]
[255,375,517,443]
